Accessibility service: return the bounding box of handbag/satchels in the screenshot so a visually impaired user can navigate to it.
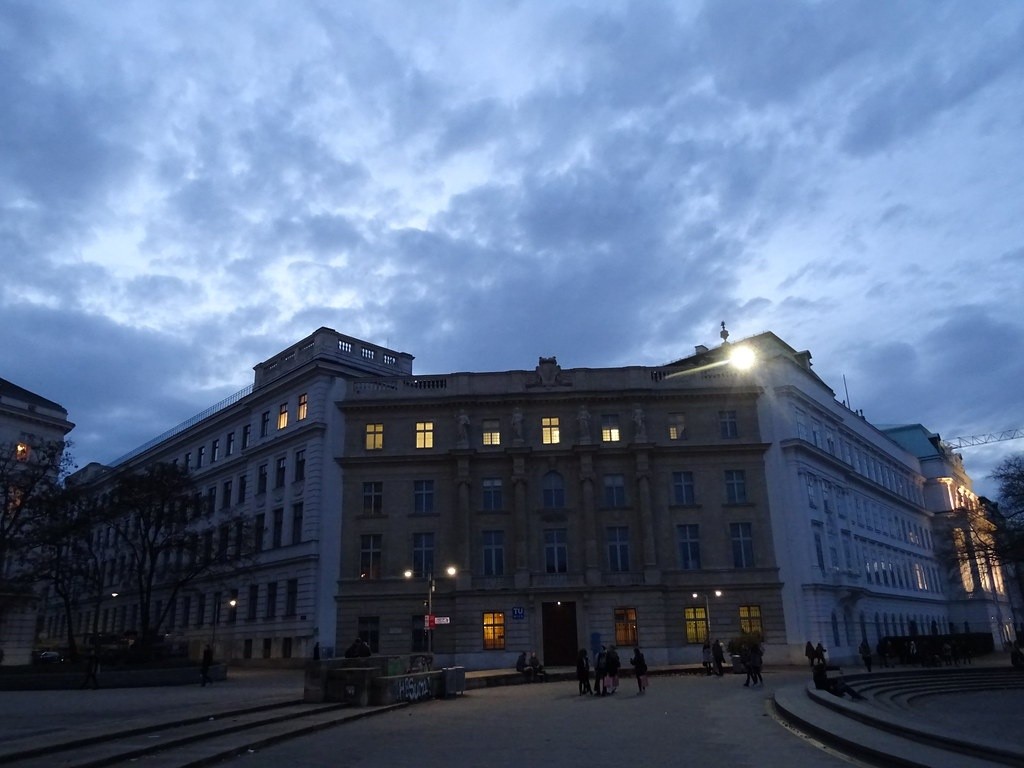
[639,675,648,688]
[604,675,612,688]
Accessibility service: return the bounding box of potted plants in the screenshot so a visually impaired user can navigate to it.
[725,631,766,674]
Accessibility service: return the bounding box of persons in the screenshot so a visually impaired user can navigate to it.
[593,645,621,697]
[529,652,546,675]
[576,648,593,696]
[702,640,714,676]
[511,406,527,435]
[516,651,533,673]
[312,641,321,659]
[858,638,872,673]
[875,638,973,668]
[805,641,815,668]
[344,637,371,658]
[575,404,591,437]
[712,639,726,676]
[1004,638,1024,669]
[742,643,764,689]
[457,408,471,441]
[200,644,214,687]
[631,403,647,435]
[815,643,826,664]
[630,648,647,695]
